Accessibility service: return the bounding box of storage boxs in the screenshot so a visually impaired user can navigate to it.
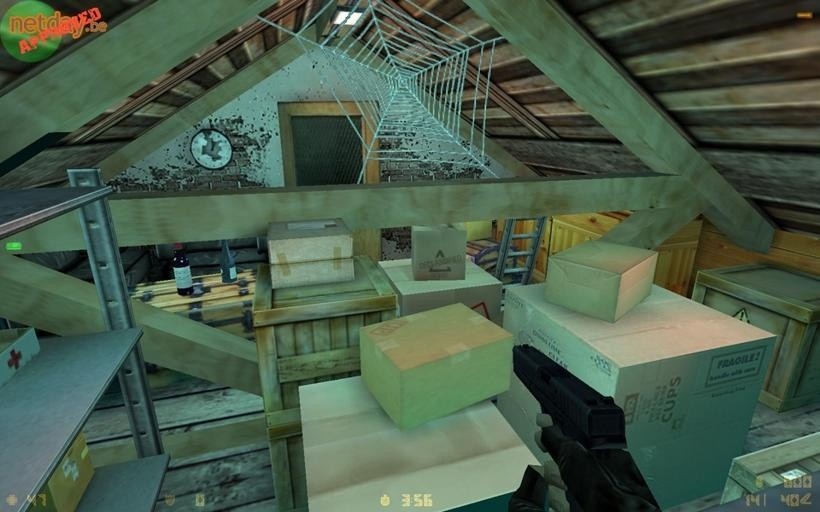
[26,219,820,512]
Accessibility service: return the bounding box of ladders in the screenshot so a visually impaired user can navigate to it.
[496,216,547,306]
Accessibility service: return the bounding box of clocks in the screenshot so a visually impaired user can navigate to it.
[190,128,234,169]
